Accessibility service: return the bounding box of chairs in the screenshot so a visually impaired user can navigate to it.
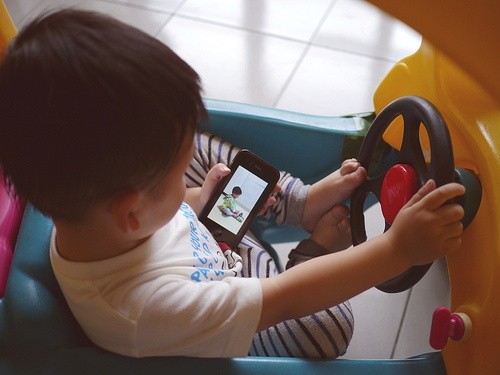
[0,96,211,375]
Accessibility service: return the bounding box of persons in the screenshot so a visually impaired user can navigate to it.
[222,186,243,219]
[0,8,466,358]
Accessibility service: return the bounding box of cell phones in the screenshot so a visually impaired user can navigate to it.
[198,148,280,251]
